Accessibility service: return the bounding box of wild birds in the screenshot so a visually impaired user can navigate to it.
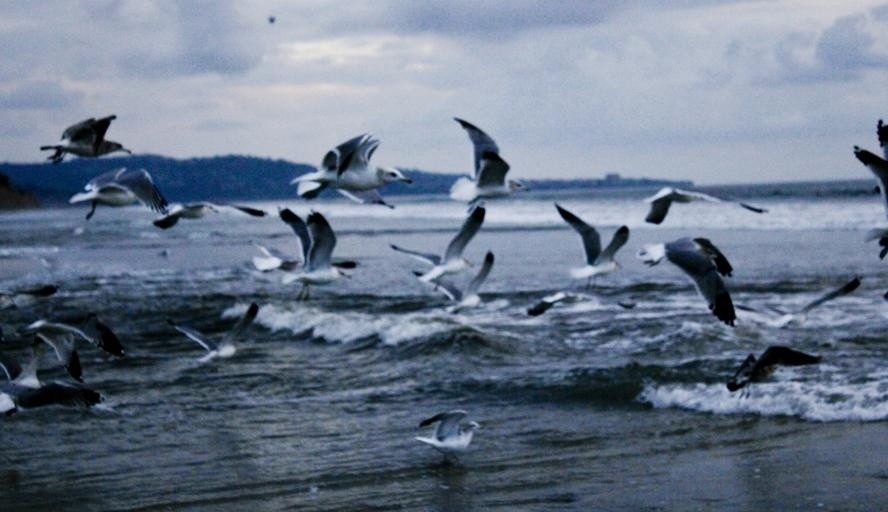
[553,202,630,287]
[167,303,259,363]
[253,117,532,315]
[727,346,822,391]
[41,115,132,164]
[526,293,636,316]
[152,201,266,229]
[646,187,768,225]
[412,411,482,461]
[766,277,861,330]
[69,167,168,219]
[854,120,888,259]
[0,286,125,417]
[639,238,739,328]
